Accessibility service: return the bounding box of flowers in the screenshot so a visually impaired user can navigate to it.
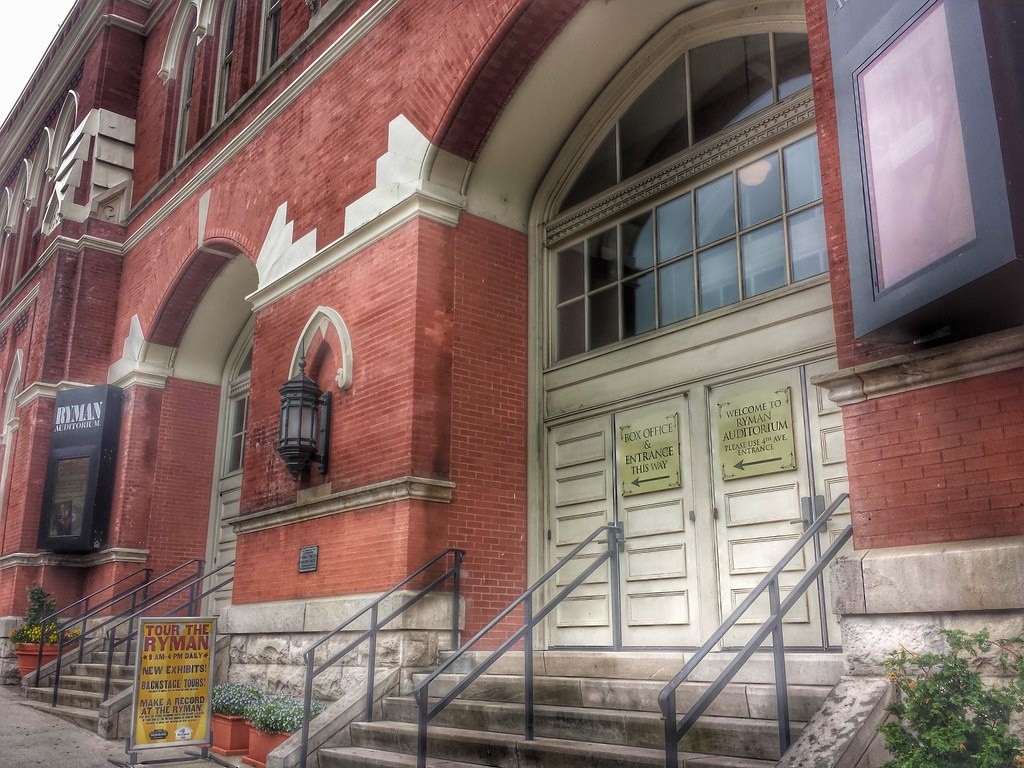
[244,691,327,734]
[8,623,82,643]
[211,681,263,716]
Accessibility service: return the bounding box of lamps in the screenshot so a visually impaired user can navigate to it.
[738,158,770,187]
[275,337,332,484]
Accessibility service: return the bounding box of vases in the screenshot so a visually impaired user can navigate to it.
[241,721,293,768]
[13,641,79,680]
[206,712,249,756]
